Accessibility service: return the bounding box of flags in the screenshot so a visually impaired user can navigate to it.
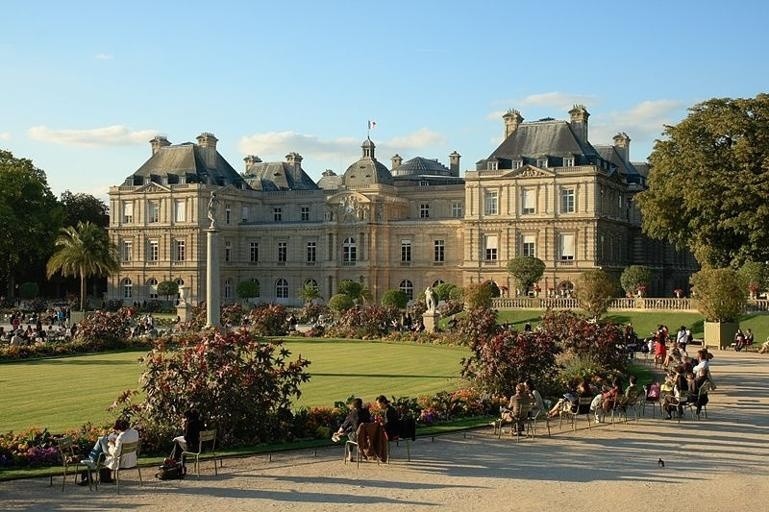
[368,119,376,130]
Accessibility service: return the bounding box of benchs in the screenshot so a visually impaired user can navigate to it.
[732,338,756,350]
[689,338,706,350]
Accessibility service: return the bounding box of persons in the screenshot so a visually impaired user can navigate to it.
[206,190,223,229]
[133,313,159,338]
[424,285,437,313]
[498,320,768,436]
[332,397,371,463]
[375,395,402,441]
[80,419,139,472]
[2,306,77,349]
[500,286,685,309]
[166,408,200,461]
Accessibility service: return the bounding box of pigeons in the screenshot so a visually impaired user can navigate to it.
[658,458,664,469]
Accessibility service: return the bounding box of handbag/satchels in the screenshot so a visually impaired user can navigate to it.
[157,465,179,480]
[647,384,658,401]
[82,471,93,483]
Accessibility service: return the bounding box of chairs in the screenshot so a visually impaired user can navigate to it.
[594,396,620,426]
[382,415,411,461]
[664,391,693,418]
[615,396,628,423]
[93,438,144,495]
[627,388,646,421]
[58,436,96,492]
[527,390,552,439]
[180,428,219,480]
[345,422,382,469]
[493,398,534,443]
[642,384,664,419]
[75,423,105,486]
[690,378,713,420]
[558,390,580,434]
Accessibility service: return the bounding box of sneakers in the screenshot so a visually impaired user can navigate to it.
[346,457,353,462]
[665,416,672,420]
[512,433,521,436]
